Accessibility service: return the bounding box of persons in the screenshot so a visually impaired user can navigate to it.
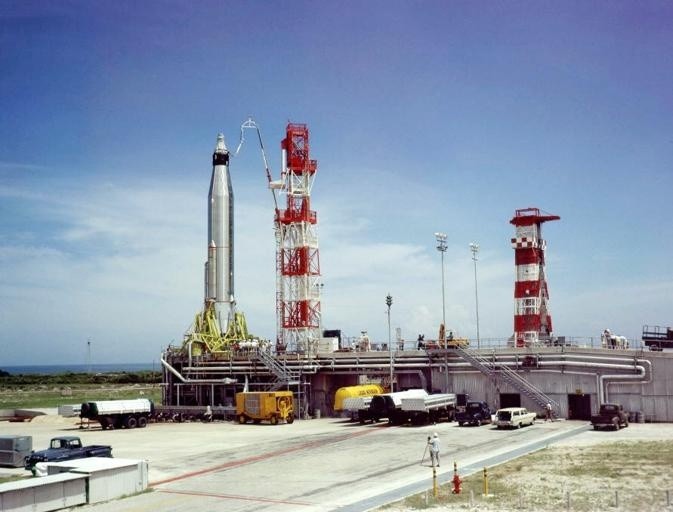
[428,433,439,467]
[230,340,272,356]
[545,401,554,422]
[447,332,453,342]
[418,334,425,350]
[602,328,627,349]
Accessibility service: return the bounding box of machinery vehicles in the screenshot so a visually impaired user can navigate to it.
[438,323,469,349]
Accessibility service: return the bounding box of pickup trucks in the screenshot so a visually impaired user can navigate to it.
[23,436,112,475]
[591,403,630,430]
[642,325,673,351]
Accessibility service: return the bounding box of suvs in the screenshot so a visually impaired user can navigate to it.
[494,407,537,429]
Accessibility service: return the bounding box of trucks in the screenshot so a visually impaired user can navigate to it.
[456,400,491,426]
[235,391,295,424]
[334,385,471,425]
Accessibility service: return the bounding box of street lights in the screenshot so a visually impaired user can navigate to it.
[434,232,449,392]
[469,240,480,354]
[386,295,395,391]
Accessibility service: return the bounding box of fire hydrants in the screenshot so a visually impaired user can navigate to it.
[452,475,462,494]
